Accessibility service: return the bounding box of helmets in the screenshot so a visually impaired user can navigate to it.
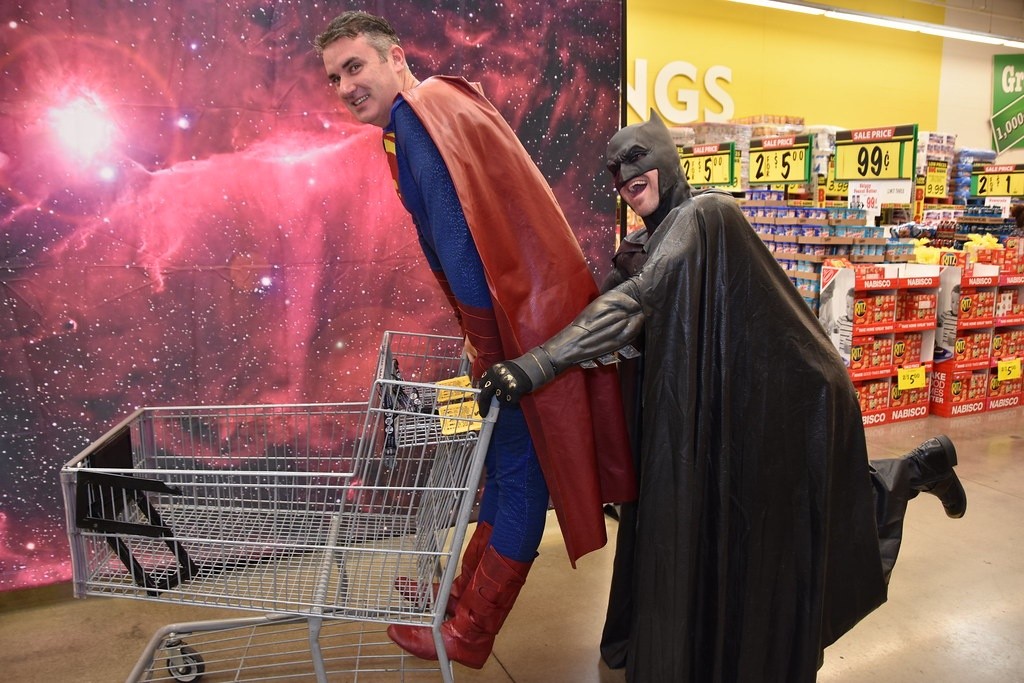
[605,106,681,213]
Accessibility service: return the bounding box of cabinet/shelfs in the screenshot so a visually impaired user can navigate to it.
[622,116,1024,428]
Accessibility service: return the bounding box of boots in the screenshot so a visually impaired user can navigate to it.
[382,544,534,671]
[394,521,496,620]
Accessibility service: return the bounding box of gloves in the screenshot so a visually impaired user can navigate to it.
[472,359,533,420]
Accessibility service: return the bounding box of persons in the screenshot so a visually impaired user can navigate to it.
[309,6,644,668]
[476,106,967,681]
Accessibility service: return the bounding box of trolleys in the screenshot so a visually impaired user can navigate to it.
[61,328,504,683]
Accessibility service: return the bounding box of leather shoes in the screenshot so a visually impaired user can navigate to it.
[901,433,968,521]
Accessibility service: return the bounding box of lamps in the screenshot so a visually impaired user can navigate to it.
[773,0,1024,63]
